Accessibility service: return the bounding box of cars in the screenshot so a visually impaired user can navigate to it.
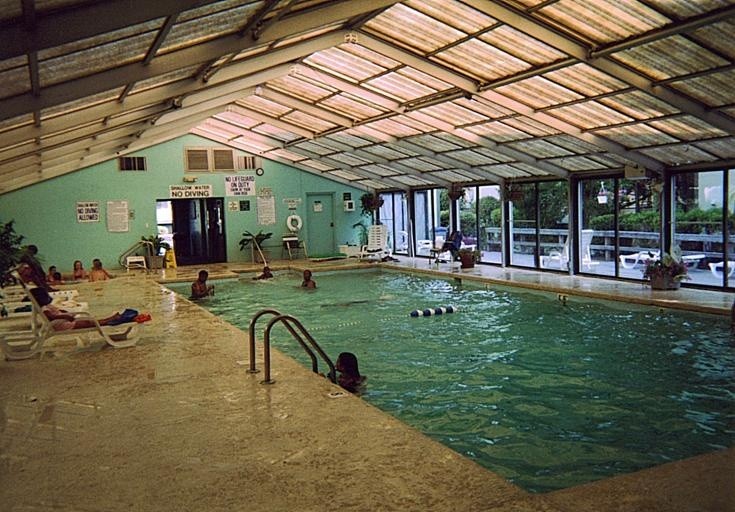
[459,234,478,245]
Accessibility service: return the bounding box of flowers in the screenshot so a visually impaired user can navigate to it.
[639,250,694,283]
[597,189,608,194]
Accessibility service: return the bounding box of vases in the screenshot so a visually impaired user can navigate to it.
[648,271,681,290]
[598,197,612,204]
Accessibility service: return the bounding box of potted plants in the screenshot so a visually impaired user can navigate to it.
[498,181,526,201]
[439,181,466,201]
[141,237,172,268]
[240,229,273,264]
[360,193,384,217]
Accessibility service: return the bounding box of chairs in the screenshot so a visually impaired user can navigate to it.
[542,228,596,271]
[280,232,309,261]
[0,270,143,360]
[358,225,479,268]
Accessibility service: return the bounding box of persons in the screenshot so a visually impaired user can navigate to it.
[19,245,110,292]
[30,288,120,331]
[318,353,365,393]
[192,271,214,298]
[296,270,315,288]
[253,267,273,280]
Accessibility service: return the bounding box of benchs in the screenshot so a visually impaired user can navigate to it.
[620,249,704,269]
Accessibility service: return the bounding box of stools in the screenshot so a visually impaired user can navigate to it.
[708,259,735,277]
[123,256,146,271]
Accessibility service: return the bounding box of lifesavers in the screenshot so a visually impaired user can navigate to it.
[287,215,303,232]
